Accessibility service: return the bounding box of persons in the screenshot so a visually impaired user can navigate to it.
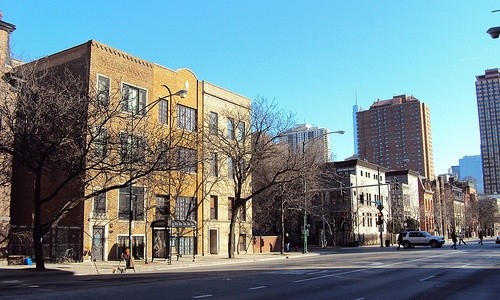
[459,232,466,245]
[478,230,483,245]
[451,230,458,249]
[123,249,130,267]
[397,232,403,250]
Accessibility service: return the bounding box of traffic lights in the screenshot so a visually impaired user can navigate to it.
[394,177,400,191]
[378,212,382,217]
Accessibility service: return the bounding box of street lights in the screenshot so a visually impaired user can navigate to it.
[377,158,410,248]
[126,89,188,269]
[302,130,347,253]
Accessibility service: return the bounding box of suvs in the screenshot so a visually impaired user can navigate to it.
[397,230,445,249]
